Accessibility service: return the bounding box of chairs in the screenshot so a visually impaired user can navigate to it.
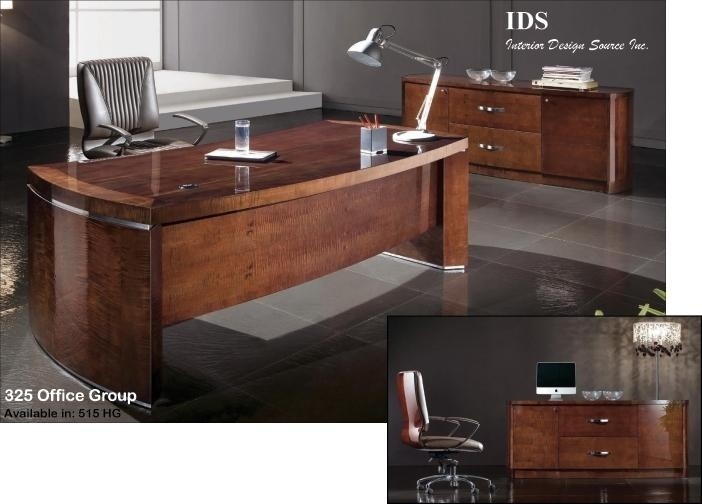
[77,57,209,159]
[396,370,496,496]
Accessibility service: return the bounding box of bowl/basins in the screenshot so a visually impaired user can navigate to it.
[603,391,623,400]
[489,70,516,82]
[466,68,491,82]
[582,391,602,401]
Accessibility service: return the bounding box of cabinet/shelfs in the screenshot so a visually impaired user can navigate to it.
[449,76,542,185]
[401,74,449,136]
[639,400,690,479]
[557,400,639,479]
[542,85,635,195]
[506,400,557,479]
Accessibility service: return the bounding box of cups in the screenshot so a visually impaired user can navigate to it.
[235,120,249,155]
[235,166,249,192]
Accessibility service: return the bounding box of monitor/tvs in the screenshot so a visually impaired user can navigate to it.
[535,361,577,401]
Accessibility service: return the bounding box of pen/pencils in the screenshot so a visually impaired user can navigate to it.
[374,114,379,128]
[359,116,368,128]
[364,114,372,129]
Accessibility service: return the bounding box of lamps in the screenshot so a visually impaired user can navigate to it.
[347,24,450,146]
[632,322,682,400]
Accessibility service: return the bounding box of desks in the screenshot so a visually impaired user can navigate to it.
[27,120,470,411]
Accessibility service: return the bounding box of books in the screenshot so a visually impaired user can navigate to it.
[541,64,601,89]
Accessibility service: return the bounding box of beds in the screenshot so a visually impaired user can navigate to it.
[69,69,323,132]
